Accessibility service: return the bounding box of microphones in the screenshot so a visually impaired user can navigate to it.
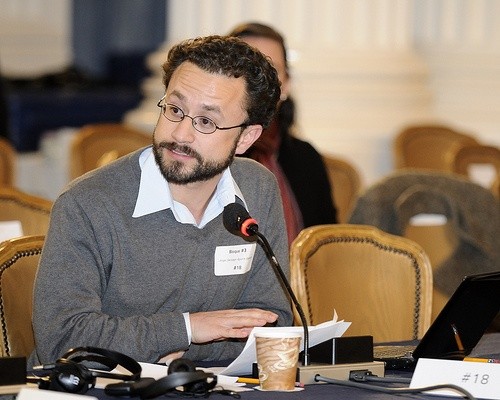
[223,202,309,365]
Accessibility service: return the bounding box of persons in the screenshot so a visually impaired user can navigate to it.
[0,0,171,152]
[27,37,294,374]
[227,23,340,247]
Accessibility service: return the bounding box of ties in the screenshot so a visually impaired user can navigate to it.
[262,157,301,252]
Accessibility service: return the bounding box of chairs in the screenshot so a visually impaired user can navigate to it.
[68,126,153,180]
[290,125,500,358]
[1,185,52,357]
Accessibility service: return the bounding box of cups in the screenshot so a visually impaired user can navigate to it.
[255,333,302,390]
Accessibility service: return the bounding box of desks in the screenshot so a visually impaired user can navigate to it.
[0,357,500,399]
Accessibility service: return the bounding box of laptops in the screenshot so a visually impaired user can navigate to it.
[371,270,500,367]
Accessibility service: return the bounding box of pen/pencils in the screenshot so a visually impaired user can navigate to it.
[237,375,305,388]
[463,358,498,363]
[451,320,466,357]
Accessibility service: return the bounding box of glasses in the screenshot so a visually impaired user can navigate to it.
[155,93,247,133]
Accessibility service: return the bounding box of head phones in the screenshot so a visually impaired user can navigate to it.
[53,346,142,394]
[132,357,219,398]
